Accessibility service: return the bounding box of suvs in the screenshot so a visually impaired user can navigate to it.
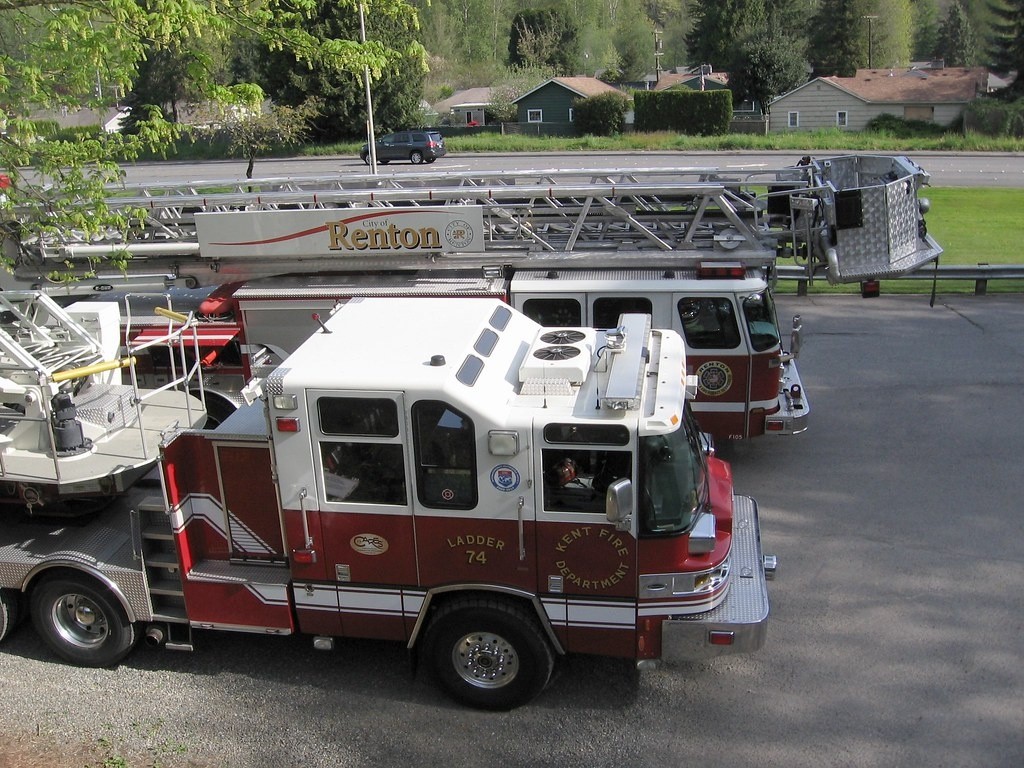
[360,131,447,166]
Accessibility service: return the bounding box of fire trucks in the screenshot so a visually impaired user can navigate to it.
[0,156,942,472]
[0,291,777,713]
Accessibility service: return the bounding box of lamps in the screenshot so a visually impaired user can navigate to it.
[764,112,770,120]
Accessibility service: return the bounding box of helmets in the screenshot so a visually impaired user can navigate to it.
[678,297,701,324]
[745,294,764,321]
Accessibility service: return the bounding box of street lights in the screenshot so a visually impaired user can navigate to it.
[861,15,878,69]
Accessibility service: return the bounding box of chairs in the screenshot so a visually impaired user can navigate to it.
[712,297,762,321]
[361,399,403,473]
[327,442,389,500]
[681,322,723,348]
[542,448,605,509]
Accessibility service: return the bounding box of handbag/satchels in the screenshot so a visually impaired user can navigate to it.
[548,459,578,487]
